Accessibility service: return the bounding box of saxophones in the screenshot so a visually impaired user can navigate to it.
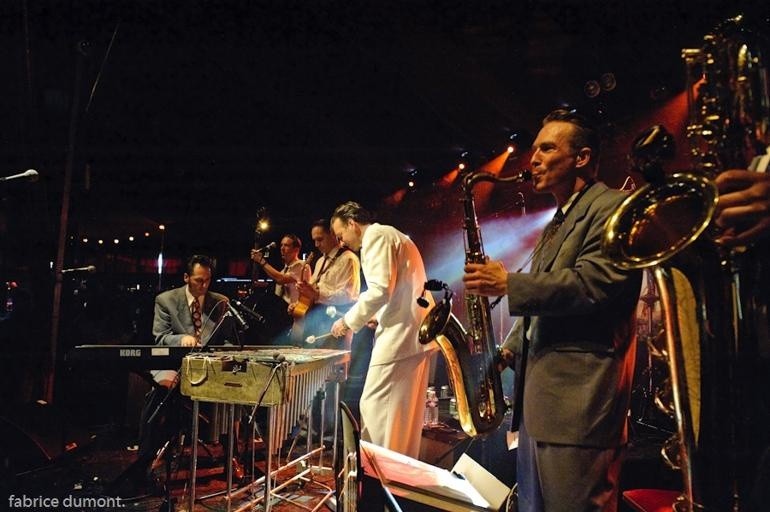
[416,170,531,438]
[602,15,768,512]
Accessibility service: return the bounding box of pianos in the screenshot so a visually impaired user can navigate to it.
[73,345,303,370]
[180,346,352,456]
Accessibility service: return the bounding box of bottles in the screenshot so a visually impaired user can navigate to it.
[423,385,458,426]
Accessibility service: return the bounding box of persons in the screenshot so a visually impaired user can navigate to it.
[248,233,312,313]
[329,202,434,467]
[461,109,644,512]
[285,218,363,389]
[121,253,246,495]
[713,169,770,248]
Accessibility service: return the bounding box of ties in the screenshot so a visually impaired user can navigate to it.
[192,298,201,344]
[541,211,564,245]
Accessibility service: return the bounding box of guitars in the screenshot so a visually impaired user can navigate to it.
[289,252,314,347]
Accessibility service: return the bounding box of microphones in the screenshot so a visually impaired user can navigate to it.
[230,296,266,326]
[226,300,246,329]
[255,241,276,255]
